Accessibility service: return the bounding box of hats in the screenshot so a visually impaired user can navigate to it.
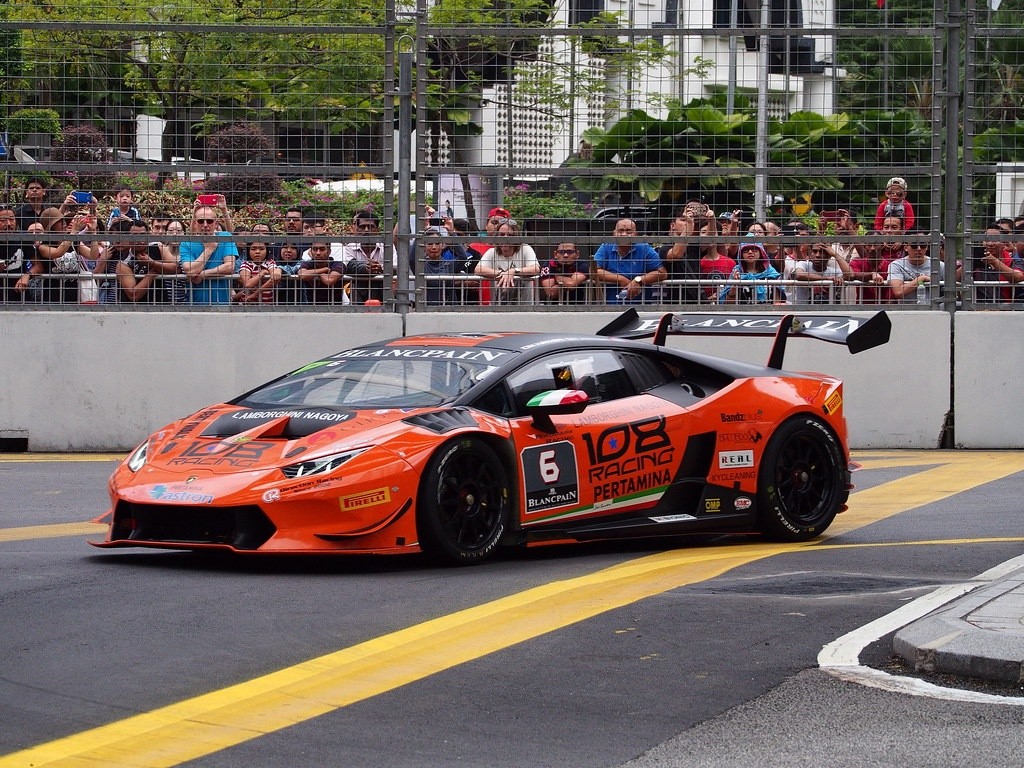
[717,212,733,222]
[928,232,945,243]
[40,207,75,231]
[886,177,907,191]
[487,207,511,220]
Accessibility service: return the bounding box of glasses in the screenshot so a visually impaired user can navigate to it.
[557,250,578,255]
[358,224,378,229]
[168,227,184,234]
[195,219,217,224]
[1004,229,1013,234]
[887,190,904,197]
[908,242,929,249]
[315,225,323,228]
[498,218,517,229]
[742,247,761,253]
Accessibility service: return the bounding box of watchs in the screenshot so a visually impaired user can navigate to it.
[634,277,642,286]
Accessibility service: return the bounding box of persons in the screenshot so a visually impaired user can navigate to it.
[15,177,1024,306]
[298,234,346,305]
[107,183,140,230]
[849,231,892,304]
[239,243,277,304]
[874,177,914,251]
[474,222,539,306]
[179,207,239,304]
[0,206,43,301]
[594,219,667,304]
[717,233,784,304]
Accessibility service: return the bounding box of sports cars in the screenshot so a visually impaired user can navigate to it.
[87,307,893,568]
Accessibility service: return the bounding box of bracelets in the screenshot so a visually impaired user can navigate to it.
[224,212,229,216]
[26,271,33,280]
[513,267,519,276]
[729,295,736,298]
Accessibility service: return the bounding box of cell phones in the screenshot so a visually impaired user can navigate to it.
[73,192,93,203]
[688,205,707,216]
[823,211,841,222]
[198,195,218,204]
[430,219,445,226]
[738,211,756,221]
[781,226,799,235]
[974,246,987,258]
